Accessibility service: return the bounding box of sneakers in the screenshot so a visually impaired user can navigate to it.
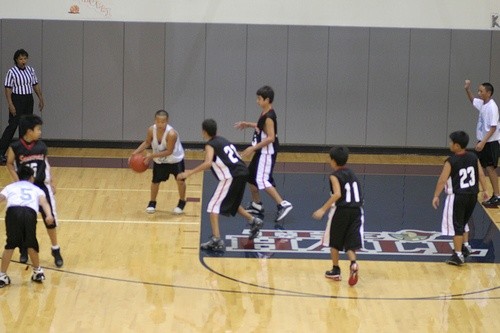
[174,201,187,213]
[481,192,500,209]
[0,275,9,289]
[325,266,342,281]
[147,201,156,212]
[51,249,64,268]
[31,269,45,281]
[244,202,265,220]
[348,264,359,284]
[247,216,263,240]
[200,238,226,251]
[274,199,293,223]
[448,251,465,265]
[452,242,472,256]
[0,152,8,165]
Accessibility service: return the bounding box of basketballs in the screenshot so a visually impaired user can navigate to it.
[129,152,147,173]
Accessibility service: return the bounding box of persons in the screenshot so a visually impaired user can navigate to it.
[311,144,365,285]
[177,118,264,252]
[8,114,63,266]
[0,166,46,286]
[127,109,187,214]
[465,79,500,208]
[0,48,44,165]
[432,130,488,265]
[233,84,293,221]
